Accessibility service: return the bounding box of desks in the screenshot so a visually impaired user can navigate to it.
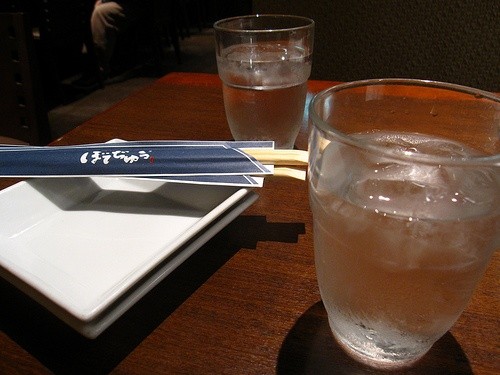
[0,71,500,375]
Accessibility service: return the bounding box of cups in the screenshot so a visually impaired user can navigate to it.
[213,15,314,150]
[309,78,500,370]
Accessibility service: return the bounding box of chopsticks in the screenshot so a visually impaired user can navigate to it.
[242,148,310,180]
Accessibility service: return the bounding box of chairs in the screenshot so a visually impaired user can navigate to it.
[6,0,217,105]
[250,0,500,93]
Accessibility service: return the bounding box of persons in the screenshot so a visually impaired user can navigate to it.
[71,0,140,89]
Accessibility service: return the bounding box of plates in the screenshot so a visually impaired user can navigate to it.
[0,139,259,339]
